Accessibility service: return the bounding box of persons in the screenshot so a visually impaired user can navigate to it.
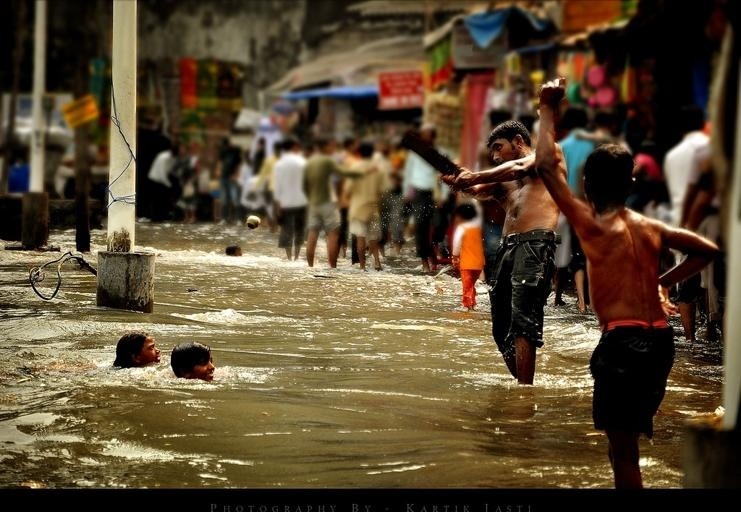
[254,109,532,310]
[551,105,677,312]
[440,120,567,388]
[661,107,722,340]
[114,330,162,370]
[169,341,215,383]
[534,74,719,488]
[149,136,268,224]
